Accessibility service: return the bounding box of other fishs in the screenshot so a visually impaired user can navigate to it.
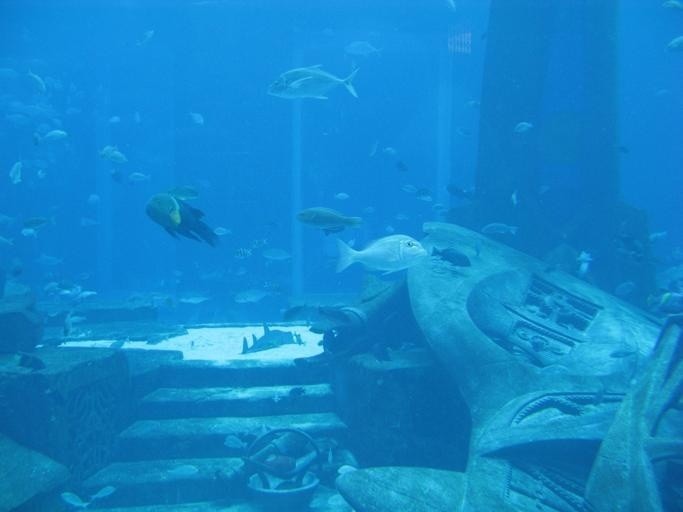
[0,249,97,372]
[186,110,206,128]
[120,268,195,347]
[99,111,148,185]
[3,70,70,185]
[3,189,107,242]
[142,187,227,249]
